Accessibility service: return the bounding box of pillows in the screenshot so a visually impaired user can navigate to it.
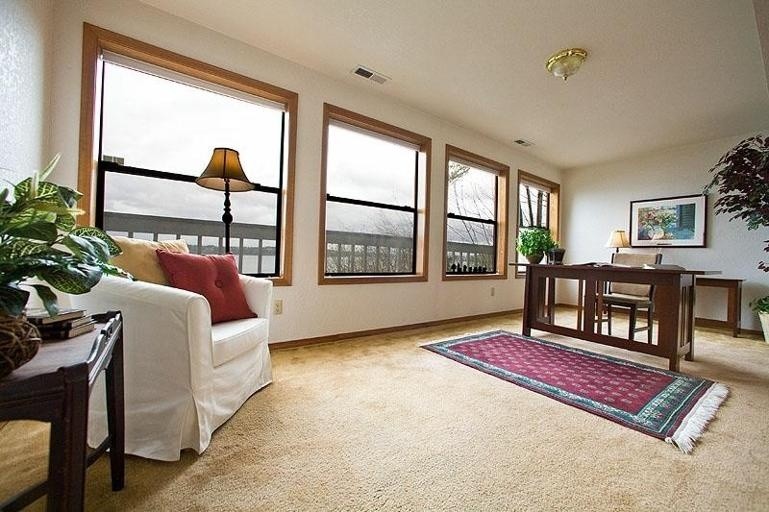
[153,245,266,326]
[102,234,192,289]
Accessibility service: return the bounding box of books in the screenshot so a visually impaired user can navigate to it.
[572,260,630,269]
[642,261,685,271]
[25,306,98,344]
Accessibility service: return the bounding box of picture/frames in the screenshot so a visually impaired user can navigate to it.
[627,193,711,249]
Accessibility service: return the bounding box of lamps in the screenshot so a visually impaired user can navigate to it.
[541,41,593,84]
[196,143,262,257]
[605,229,633,253]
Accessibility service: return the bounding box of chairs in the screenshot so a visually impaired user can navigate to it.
[595,250,668,347]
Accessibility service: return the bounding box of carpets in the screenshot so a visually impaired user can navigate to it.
[415,326,735,455]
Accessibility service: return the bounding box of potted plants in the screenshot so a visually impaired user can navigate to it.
[701,132,769,358]
[510,226,556,264]
[0,144,138,379]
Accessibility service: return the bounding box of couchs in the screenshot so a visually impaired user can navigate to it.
[7,212,282,466]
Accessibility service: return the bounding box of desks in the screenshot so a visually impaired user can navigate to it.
[507,260,726,373]
[1,302,129,511]
[693,276,747,338]
